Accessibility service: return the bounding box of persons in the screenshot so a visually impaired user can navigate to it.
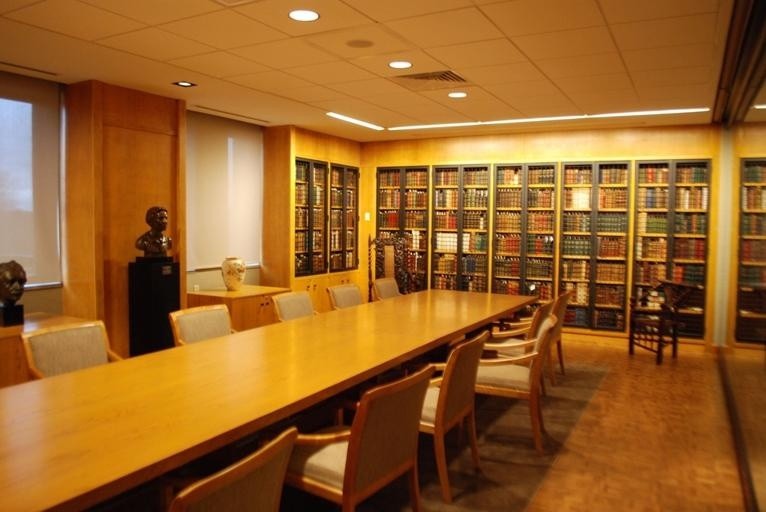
[135,206,173,258]
[0,261,28,305]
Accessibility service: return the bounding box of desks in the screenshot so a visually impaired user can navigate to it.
[0,289,537,511]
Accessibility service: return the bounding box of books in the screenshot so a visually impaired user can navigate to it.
[379,171,400,239]
[462,168,489,292]
[671,167,709,334]
[434,168,457,290]
[635,168,669,331]
[296,165,356,272]
[405,169,427,293]
[525,167,556,312]
[563,168,592,324]
[595,167,628,328]
[493,168,522,317]
[739,166,765,339]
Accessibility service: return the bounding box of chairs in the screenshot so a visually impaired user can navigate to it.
[335,330,490,505]
[271,290,319,322]
[167,303,238,346]
[19,319,124,379]
[372,278,404,301]
[484,302,555,397]
[132,426,300,511]
[515,290,573,386]
[259,364,437,512]
[427,313,558,450]
[628,280,693,364]
[325,283,366,310]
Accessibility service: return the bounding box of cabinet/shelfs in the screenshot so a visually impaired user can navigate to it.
[0,312,83,387]
[263,155,362,314]
[187,285,290,330]
[734,157,765,345]
